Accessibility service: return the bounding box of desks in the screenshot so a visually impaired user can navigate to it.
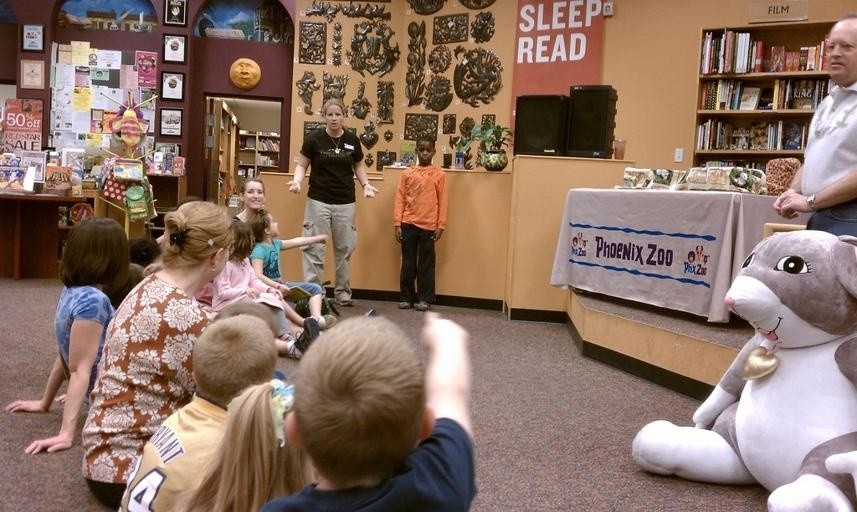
[549,188,813,325]
[0,193,96,281]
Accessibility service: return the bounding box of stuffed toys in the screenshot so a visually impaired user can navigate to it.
[631,228,856,511]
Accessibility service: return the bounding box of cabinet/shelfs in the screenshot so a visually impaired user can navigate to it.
[235,127,281,179]
[690,17,840,173]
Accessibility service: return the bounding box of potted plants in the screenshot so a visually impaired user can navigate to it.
[456,124,514,171]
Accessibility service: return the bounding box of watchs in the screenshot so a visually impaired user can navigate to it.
[807,193,818,210]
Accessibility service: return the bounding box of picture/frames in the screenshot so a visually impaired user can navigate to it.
[159,107,184,137]
[20,24,45,54]
[162,32,187,65]
[20,59,45,90]
[160,71,185,101]
[163,0,189,27]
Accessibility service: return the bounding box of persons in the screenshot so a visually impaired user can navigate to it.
[394,135,448,310]
[264,308,478,511]
[6,179,337,511]
[287,99,380,306]
[773,13,856,240]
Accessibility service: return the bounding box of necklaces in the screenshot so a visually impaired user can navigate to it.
[325,128,343,154]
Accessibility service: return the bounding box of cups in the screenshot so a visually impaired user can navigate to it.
[443,153,452,168]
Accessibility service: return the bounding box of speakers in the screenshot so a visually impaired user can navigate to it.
[567,84,617,159]
[512,94,569,156]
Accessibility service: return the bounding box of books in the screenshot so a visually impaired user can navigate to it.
[697,29,836,150]
[206,114,280,178]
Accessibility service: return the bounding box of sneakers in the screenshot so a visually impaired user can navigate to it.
[335,293,353,306]
[399,300,431,311]
[279,315,337,359]
